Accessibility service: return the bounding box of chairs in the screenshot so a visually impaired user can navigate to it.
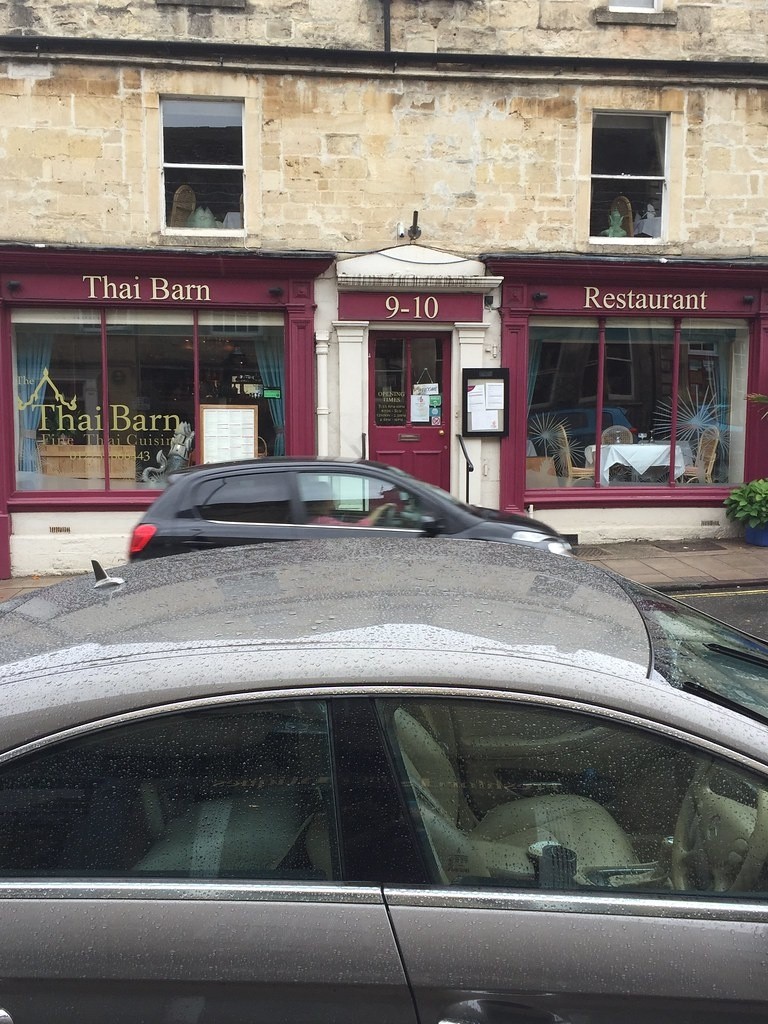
[394,707,644,889]
[602,426,634,444]
[680,427,722,483]
[557,424,595,486]
[610,196,634,237]
[167,186,197,226]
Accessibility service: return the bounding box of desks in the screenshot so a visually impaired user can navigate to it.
[632,216,662,238]
[584,444,686,484]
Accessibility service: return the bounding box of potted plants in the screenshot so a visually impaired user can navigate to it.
[722,478,768,548]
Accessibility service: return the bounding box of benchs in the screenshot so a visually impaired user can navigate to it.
[73,769,317,874]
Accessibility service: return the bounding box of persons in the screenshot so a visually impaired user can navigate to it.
[308,483,396,527]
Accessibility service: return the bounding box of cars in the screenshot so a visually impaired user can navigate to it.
[0,537,767,1024]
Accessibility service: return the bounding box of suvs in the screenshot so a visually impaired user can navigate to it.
[128,455,579,562]
[527,406,645,466]
[681,402,741,440]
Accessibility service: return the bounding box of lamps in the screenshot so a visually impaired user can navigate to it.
[743,295,755,304]
[268,287,284,296]
[6,280,20,288]
[532,291,551,300]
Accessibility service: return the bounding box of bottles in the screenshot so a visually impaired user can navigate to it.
[615,431,621,444]
[650,430,654,443]
